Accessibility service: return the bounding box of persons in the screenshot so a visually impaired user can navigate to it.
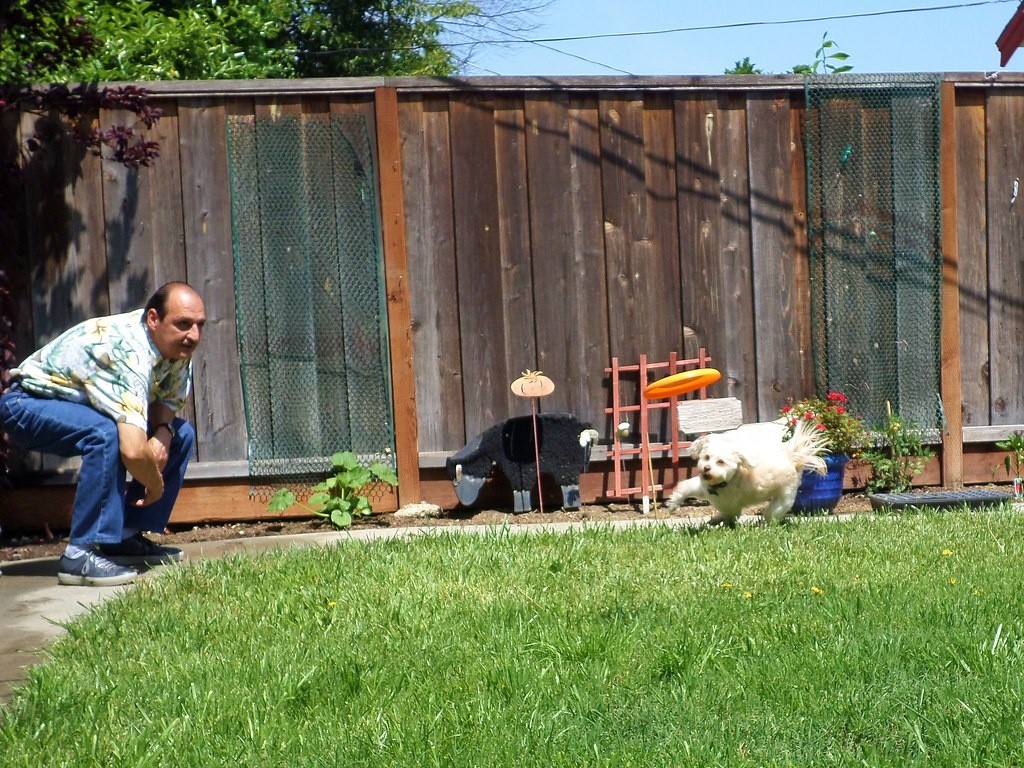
[0,280,207,587]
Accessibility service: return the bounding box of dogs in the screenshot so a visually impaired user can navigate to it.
[667,421,832,530]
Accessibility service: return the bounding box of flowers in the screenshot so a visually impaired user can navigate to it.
[780,390,873,455]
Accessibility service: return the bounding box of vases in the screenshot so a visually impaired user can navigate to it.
[789,454,852,515]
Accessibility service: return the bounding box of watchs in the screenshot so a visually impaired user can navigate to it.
[156,423,175,438]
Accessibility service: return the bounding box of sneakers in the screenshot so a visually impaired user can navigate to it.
[57,549,137,586]
[100,534,184,565]
